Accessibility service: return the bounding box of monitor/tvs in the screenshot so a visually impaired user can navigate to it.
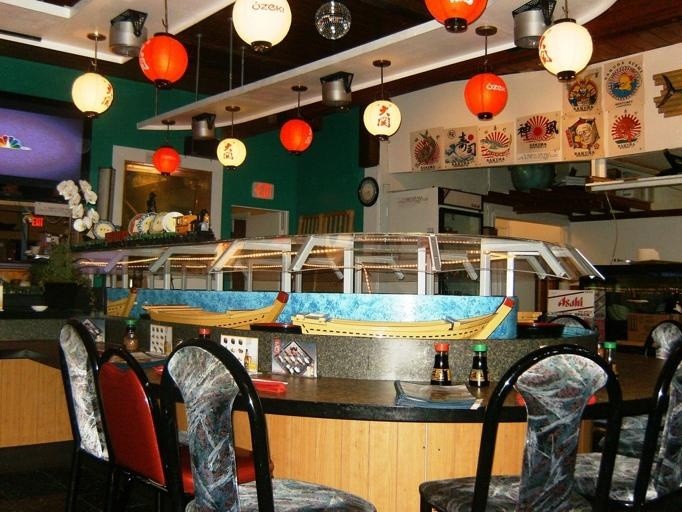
[0,91,94,205]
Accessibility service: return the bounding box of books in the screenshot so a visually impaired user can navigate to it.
[392,380,478,410]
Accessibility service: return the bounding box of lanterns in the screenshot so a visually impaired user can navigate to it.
[230,0,294,53]
[139,34,189,90]
[216,137,249,171]
[424,1,489,34]
[538,19,595,80]
[72,73,115,119]
[280,120,313,157]
[152,146,182,177]
[462,73,508,121]
[363,98,402,141]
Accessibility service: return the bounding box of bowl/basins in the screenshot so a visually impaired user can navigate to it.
[517,322,565,339]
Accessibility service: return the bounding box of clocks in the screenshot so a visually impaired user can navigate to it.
[358,177,378,207]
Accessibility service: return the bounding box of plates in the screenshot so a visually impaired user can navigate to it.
[92,220,117,240]
[127,211,183,234]
[31,305,48,312]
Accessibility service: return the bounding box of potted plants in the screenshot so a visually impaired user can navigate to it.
[38,243,80,313]
[3,282,45,314]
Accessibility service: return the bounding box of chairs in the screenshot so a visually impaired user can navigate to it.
[418,344,623,512]
[575,347,682,512]
[592,320,682,429]
[545,316,590,337]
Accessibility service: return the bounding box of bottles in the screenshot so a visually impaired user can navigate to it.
[430,343,452,385]
[470,344,490,386]
[198,328,212,341]
[601,342,620,382]
[176,208,210,231]
[122,320,139,354]
[26,233,65,262]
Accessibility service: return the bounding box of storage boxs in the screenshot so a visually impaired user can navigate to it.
[547,289,606,320]
